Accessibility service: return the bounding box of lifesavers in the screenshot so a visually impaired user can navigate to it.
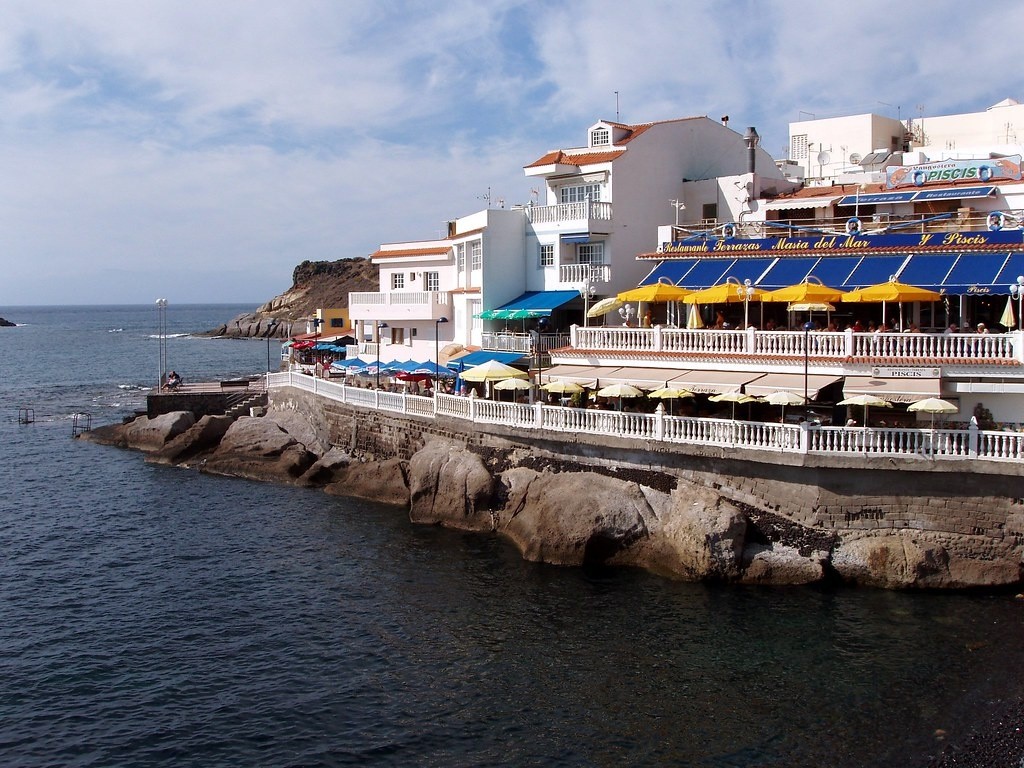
[845,218,862,236]
[912,170,926,186]
[986,211,1005,231]
[976,165,992,182]
[722,224,736,239]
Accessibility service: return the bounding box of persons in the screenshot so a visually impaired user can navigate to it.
[553,398,1024,437]
[161,370,181,391]
[343,376,456,397]
[623,309,992,334]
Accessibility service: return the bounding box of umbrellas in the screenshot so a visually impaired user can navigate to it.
[705,390,756,420]
[647,387,695,416]
[458,359,528,402]
[595,384,644,410]
[587,298,622,318]
[836,395,893,426]
[494,377,534,402]
[285,339,585,375]
[906,397,960,429]
[538,381,584,406]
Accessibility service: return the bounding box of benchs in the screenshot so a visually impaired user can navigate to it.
[168,377,184,392]
[220,381,249,391]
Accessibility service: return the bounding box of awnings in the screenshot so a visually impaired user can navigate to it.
[618,251,1023,304]
[530,363,767,397]
[999,295,1016,334]
[473,289,580,321]
[761,185,997,211]
[446,349,527,374]
[843,377,941,405]
[744,373,844,400]
[758,389,810,425]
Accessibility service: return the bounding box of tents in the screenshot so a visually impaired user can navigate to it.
[614,282,942,307]
